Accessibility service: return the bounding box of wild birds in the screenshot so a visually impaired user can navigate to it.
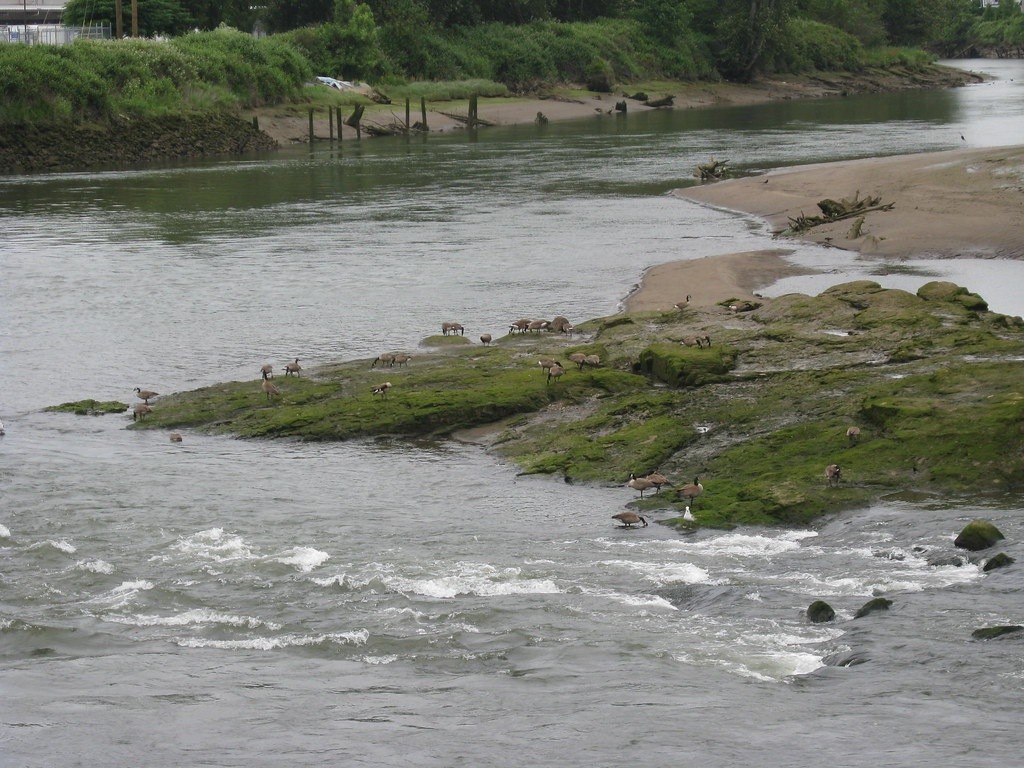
[612,426,861,528]
[959,132,967,141]
[132,294,711,423]
[763,179,768,183]
